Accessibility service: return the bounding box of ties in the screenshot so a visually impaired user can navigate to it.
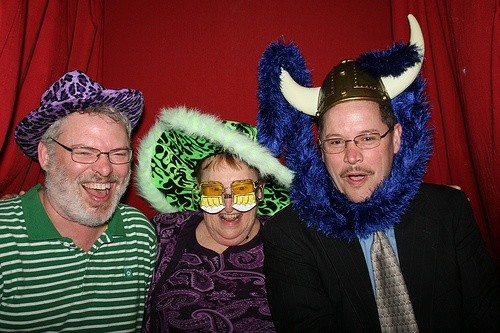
[371,230,420,333]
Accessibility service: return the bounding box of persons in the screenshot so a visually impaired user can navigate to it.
[256,14,500,333]
[0,70,159,333]
[0,103,471,333]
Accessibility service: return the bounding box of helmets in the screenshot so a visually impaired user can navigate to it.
[279,14,424,119]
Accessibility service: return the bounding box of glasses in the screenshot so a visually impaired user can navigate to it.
[319,128,391,154]
[50,137,134,165]
[199,179,265,213]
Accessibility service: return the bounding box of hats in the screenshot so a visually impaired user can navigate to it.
[133,106,294,217]
[14,70,144,162]
